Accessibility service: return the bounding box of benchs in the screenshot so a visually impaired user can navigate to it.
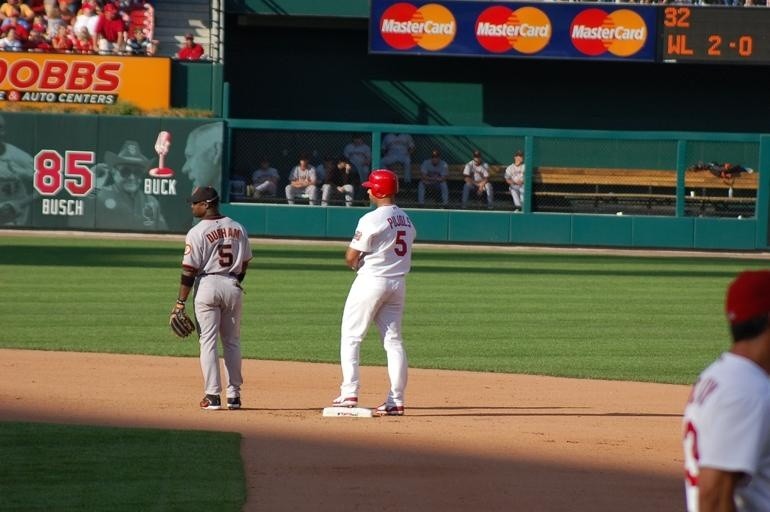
[390,164,758,211]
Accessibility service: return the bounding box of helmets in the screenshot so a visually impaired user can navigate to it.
[361,169,397,198]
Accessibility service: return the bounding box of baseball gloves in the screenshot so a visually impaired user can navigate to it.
[170,307,194,338]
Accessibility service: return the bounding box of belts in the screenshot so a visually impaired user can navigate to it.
[229,272,239,279]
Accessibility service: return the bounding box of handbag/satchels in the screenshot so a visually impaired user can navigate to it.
[710,165,741,178]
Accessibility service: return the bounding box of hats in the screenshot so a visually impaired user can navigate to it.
[185,33,193,38]
[103,140,151,168]
[186,186,218,203]
[104,4,118,15]
[472,150,480,158]
[82,3,93,10]
[432,150,439,157]
[726,271,770,324]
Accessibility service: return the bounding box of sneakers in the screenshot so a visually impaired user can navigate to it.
[226,397,241,408]
[332,396,358,407]
[376,403,404,415]
[200,395,221,410]
[417,202,523,211]
[250,185,262,199]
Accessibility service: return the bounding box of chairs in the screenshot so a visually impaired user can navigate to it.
[226,180,247,203]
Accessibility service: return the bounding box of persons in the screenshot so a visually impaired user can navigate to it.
[76,140,170,230]
[246,133,526,211]
[180,121,222,199]
[682,268,770,512]
[0,0,157,57]
[171,33,204,60]
[319,169,416,414]
[170,186,252,409]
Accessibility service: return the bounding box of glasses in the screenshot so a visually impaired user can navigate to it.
[114,165,143,178]
